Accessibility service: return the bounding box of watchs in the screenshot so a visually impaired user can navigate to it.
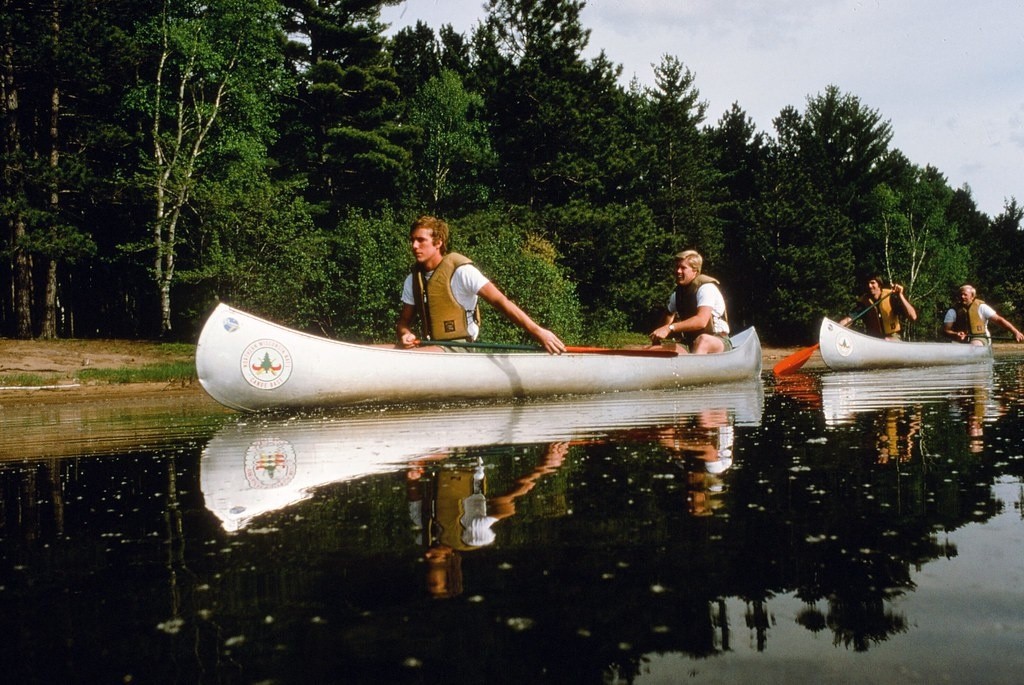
[669,322,675,332]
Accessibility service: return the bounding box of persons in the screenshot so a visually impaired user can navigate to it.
[649,250,732,355]
[396,215,567,355]
[943,285,1024,347]
[838,274,918,341]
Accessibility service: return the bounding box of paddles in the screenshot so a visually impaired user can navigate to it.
[409,339,679,359]
[963,334,1024,341]
[773,288,898,375]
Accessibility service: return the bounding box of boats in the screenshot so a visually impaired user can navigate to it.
[195,301,764,414]
[820,362,994,436]
[818,317,993,368]
[197,380,764,541]
